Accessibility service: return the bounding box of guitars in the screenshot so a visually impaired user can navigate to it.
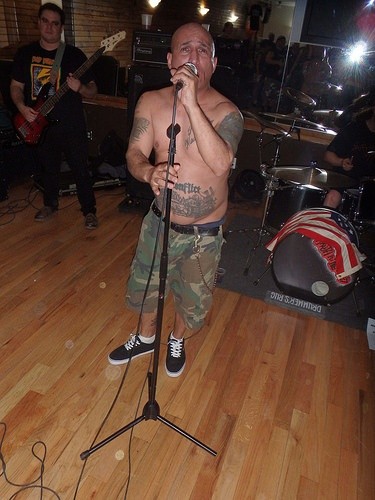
[12,28,128,146]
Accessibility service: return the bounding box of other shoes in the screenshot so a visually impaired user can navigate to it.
[34,207,59,222]
[85,213,97,229]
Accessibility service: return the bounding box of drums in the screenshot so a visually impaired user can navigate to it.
[270,207,360,305]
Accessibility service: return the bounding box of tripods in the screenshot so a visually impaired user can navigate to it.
[80,91,218,461]
[221,135,375,318]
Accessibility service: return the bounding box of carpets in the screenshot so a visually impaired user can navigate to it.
[213,213,375,330]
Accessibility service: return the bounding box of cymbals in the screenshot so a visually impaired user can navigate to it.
[262,87,344,136]
[268,168,353,188]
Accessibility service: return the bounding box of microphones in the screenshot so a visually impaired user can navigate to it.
[175,63,198,90]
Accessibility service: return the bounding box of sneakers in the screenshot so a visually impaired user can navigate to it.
[165,331,186,377]
[108,333,156,364]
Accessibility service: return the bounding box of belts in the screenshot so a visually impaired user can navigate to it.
[151,201,219,236]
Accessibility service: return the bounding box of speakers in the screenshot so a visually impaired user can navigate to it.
[213,37,250,111]
[0,136,47,199]
[126,67,174,211]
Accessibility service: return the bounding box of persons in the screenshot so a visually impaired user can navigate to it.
[104,21,246,381]
[324,104,375,208]
[8,1,100,230]
[217,19,343,124]
